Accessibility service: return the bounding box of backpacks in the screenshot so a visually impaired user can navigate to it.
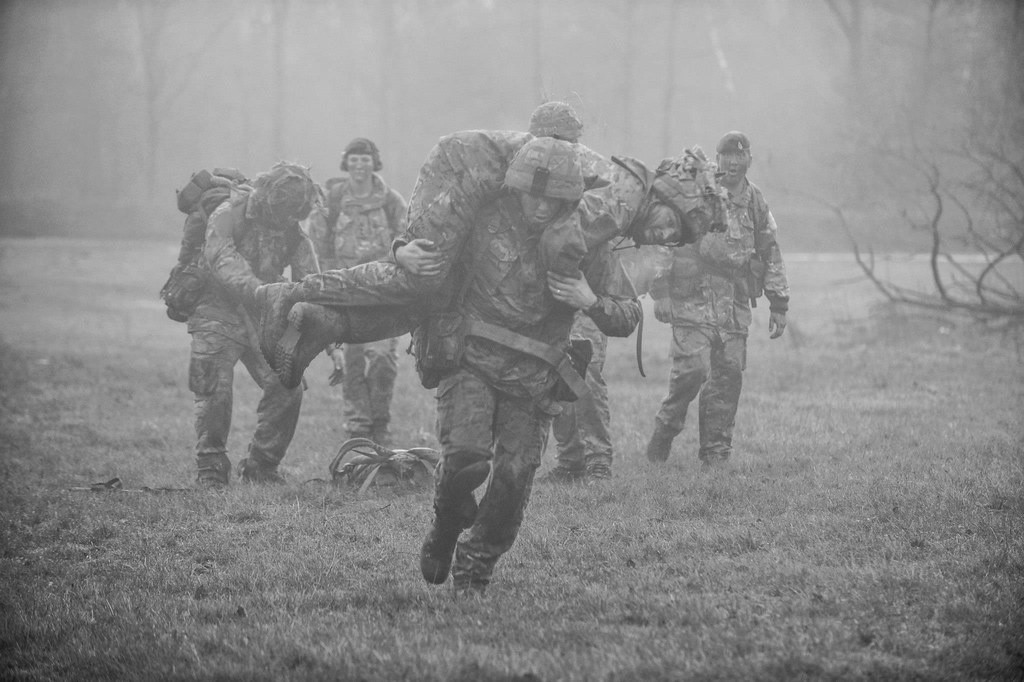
[177,187,231,265]
[330,438,440,492]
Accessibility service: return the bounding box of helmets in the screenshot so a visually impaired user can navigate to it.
[655,147,726,243]
[340,139,383,173]
[245,162,312,223]
[529,103,581,143]
[504,137,585,201]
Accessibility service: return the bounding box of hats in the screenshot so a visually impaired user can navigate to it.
[715,131,750,153]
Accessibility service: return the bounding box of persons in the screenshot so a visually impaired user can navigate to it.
[181,161,346,487]
[307,136,408,454]
[256,129,728,596]
[646,131,789,467]
[526,102,615,485]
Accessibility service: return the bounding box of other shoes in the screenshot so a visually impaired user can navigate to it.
[276,301,327,389]
[257,283,291,370]
[195,468,227,491]
[533,466,579,486]
[370,421,389,444]
[241,457,285,488]
[420,521,458,584]
[649,422,674,463]
[453,577,486,601]
[586,464,610,489]
